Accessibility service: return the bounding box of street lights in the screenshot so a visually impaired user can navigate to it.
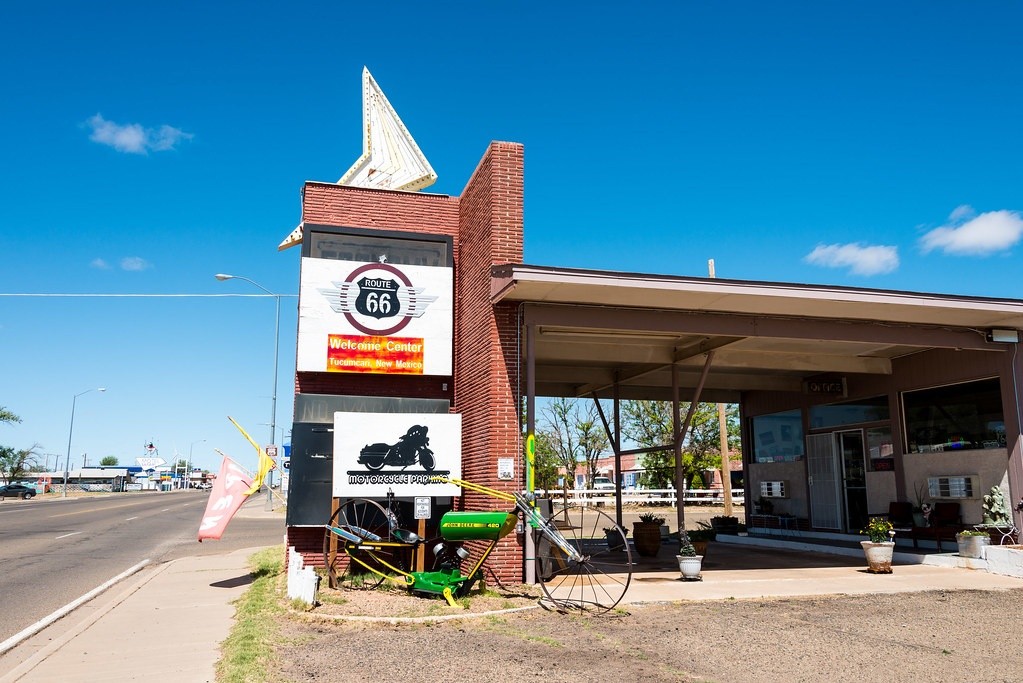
[214,273,280,511]
[190,440,206,470]
[64,388,106,496]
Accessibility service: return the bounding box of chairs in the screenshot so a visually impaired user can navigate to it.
[915,502,963,551]
[867,501,913,545]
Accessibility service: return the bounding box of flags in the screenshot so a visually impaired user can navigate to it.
[232,420,274,496]
[198,457,258,543]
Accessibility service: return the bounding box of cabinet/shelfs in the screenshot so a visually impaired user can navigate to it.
[760,481,790,499]
[928,475,980,500]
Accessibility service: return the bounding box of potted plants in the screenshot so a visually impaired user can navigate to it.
[602,526,630,552]
[687,529,708,564]
[751,500,776,528]
[710,515,739,536]
[913,482,936,527]
[633,511,662,558]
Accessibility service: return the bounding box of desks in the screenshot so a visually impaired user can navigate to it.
[535,526,583,572]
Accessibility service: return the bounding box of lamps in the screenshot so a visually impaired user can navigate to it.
[806,376,847,399]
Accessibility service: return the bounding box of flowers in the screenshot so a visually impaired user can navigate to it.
[677,520,697,556]
[859,517,894,543]
[955,530,989,537]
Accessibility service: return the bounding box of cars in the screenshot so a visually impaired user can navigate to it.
[589,477,616,497]
[0,485,36,500]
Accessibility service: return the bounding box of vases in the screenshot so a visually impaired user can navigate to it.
[956,534,991,558]
[861,541,895,573]
[676,555,703,579]
